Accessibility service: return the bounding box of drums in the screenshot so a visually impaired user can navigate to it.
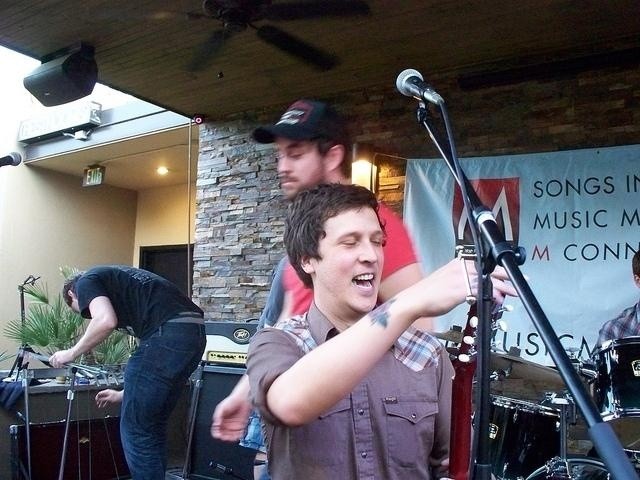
[471,394,569,480]
[519,453,611,480]
[594,335,640,421]
[623,444,640,475]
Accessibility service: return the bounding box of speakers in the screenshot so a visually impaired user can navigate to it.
[182,360,258,480]
[23,53,98,107]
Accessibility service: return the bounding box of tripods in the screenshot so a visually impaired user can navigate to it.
[8,275,53,381]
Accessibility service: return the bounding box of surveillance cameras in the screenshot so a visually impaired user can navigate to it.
[194,114,204,124]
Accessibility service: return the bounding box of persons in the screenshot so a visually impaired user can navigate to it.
[246,179,528,480]
[590,249,640,360]
[49,263,208,480]
[210,99,436,480]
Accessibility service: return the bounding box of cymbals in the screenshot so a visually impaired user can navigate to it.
[429,329,463,343]
[475,352,566,400]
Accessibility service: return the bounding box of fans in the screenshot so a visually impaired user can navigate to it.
[89,0,371,71]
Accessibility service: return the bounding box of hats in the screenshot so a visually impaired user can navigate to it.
[251,99,351,144]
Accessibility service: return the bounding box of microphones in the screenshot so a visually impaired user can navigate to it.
[395,68,445,105]
[0,151,21,168]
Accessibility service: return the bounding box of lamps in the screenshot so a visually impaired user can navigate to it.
[352,142,379,195]
